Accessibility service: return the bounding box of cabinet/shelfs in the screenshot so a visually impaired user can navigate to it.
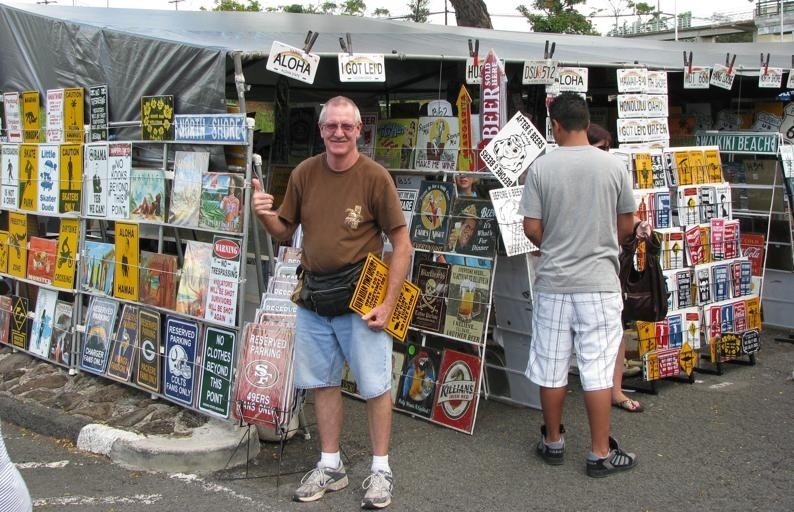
[1,117,312,442]
[224,118,791,438]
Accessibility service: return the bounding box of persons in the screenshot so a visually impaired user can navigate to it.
[133,193,162,217]
[93,162,102,194]
[252,96,412,509]
[587,124,644,413]
[218,177,242,226]
[51,314,73,362]
[67,156,73,190]
[456,175,475,197]
[121,238,130,278]
[6,159,13,182]
[450,216,478,252]
[36,309,46,346]
[61,236,69,254]
[516,91,637,477]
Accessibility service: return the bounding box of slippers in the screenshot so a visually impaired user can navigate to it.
[613,397,644,413]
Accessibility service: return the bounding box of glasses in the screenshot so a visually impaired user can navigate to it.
[325,123,355,132]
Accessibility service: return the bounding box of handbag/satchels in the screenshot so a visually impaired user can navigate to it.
[299,263,364,317]
[620,221,667,322]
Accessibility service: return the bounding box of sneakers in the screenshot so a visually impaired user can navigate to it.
[538,424,566,465]
[293,459,348,501]
[587,436,637,478]
[361,468,393,509]
